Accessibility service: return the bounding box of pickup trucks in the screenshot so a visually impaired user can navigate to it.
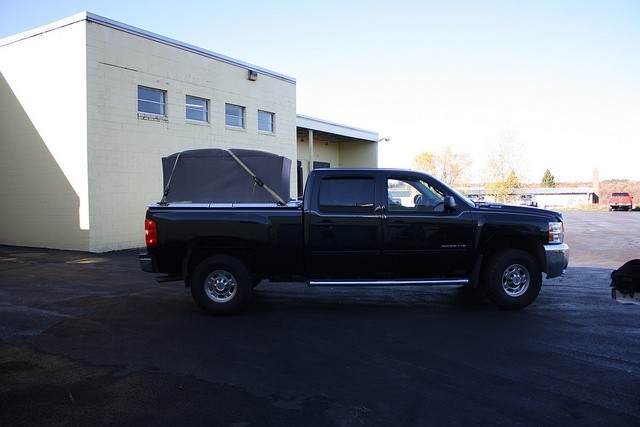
[139,167,568,317]
[517,194,537,207]
[609,192,633,212]
